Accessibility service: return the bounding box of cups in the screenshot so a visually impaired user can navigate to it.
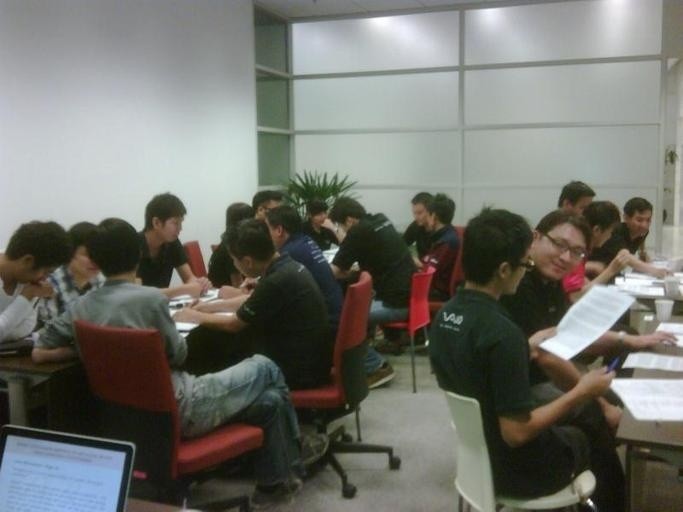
[654,300,675,322]
[663,276,680,299]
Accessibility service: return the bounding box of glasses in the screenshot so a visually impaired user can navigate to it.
[543,231,590,262]
[508,256,535,274]
[259,205,271,214]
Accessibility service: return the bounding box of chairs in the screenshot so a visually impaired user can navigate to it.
[183,240,206,278]
[74,320,264,509]
[289,272,401,498]
[383,265,435,392]
[211,244,219,251]
[429,224,466,313]
[443,390,600,512]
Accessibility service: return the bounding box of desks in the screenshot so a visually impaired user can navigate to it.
[630,297,683,314]
[617,314,683,511]
[0,287,239,427]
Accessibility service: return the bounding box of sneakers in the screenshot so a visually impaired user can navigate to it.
[363,361,395,390]
[249,478,304,512]
[289,433,330,468]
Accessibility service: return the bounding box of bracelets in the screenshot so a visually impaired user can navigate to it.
[619,328,627,349]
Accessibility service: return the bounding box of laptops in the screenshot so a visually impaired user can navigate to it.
[0,423,138,512]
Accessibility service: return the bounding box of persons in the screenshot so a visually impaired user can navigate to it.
[601,198,675,284]
[252,191,417,387]
[500,207,679,445]
[0,187,326,510]
[429,206,628,512]
[556,181,605,273]
[376,192,457,354]
[562,200,632,307]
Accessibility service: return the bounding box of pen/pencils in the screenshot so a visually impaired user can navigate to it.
[606,353,624,372]
[623,268,626,282]
